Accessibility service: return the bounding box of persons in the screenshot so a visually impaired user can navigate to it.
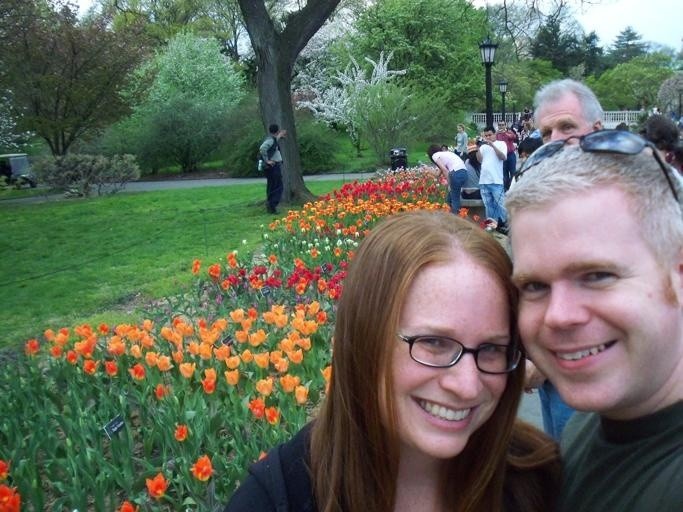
[223,210,564,511]
[426,107,543,238]
[502,129,682,511]
[615,107,683,177]
[521,77,607,445]
[260,124,287,213]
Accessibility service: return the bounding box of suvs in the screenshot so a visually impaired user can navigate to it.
[0,153,38,190]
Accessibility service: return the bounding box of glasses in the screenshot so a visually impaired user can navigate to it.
[398,334,522,374]
[515,130,679,201]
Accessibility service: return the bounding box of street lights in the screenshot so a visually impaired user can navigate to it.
[477,30,499,130]
[496,81,511,122]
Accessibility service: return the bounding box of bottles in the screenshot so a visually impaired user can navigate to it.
[257,160,262,171]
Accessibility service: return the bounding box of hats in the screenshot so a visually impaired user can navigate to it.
[467,146,478,153]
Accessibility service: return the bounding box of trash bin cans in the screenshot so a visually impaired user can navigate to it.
[389,148,408,171]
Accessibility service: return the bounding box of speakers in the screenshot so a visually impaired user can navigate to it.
[391,148,407,169]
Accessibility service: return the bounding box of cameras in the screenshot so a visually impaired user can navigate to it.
[498,130,504,133]
[476,138,487,144]
